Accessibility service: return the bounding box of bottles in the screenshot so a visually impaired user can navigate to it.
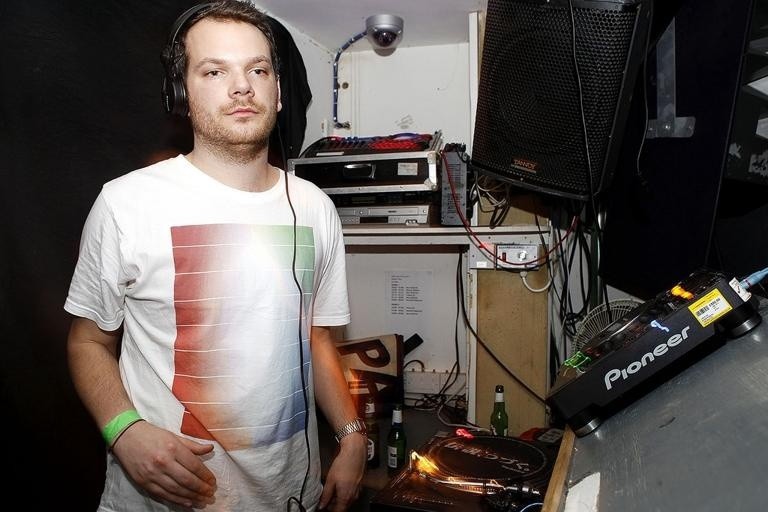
[488,384,509,436]
[386,403,407,476]
[362,396,380,468]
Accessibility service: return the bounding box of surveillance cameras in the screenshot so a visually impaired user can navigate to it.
[365,13,404,50]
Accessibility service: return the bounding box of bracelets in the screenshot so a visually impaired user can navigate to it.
[102,408,146,450]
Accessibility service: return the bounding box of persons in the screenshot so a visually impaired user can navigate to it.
[59,2,370,510]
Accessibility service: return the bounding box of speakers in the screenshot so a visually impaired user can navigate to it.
[469,1,753,219]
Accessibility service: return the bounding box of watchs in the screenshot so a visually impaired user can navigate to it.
[332,415,368,444]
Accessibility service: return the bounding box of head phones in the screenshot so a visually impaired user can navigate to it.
[161,3,281,119]
